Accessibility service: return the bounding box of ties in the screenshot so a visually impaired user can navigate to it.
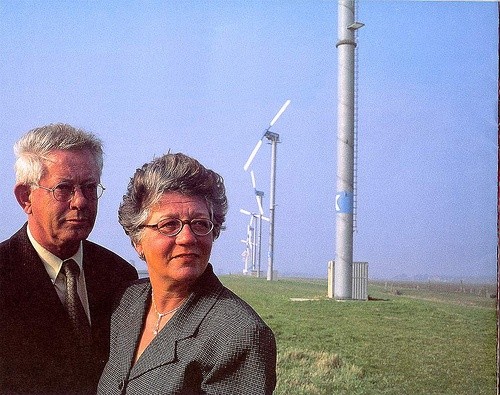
[61,259,96,373]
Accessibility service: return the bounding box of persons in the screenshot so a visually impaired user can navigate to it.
[97,151,277,395]
[0,122,140,395]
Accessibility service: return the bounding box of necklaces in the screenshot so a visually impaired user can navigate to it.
[151,287,187,337]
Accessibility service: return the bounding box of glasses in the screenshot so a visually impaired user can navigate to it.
[137,216,216,237]
[30,180,106,205]
